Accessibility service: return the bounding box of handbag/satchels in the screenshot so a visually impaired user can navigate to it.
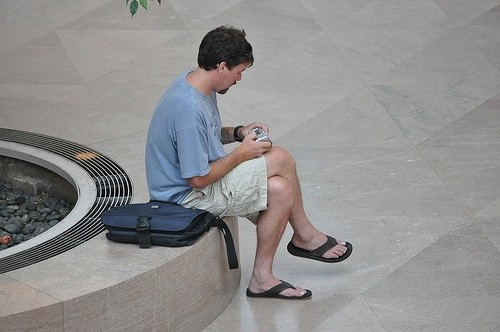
[102,200,215,248]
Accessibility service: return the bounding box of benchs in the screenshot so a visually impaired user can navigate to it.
[0,96,243,332]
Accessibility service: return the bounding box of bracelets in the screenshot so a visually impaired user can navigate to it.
[239,145,249,162]
[233,125,244,141]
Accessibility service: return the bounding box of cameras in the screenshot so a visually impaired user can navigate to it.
[249,128,272,143]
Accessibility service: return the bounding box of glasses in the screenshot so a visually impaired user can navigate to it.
[226,43,253,60]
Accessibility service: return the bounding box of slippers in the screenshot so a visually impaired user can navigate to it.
[246,279,312,300]
[288,234,353,263]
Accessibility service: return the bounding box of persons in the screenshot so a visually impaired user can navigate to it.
[145,26,353,300]
